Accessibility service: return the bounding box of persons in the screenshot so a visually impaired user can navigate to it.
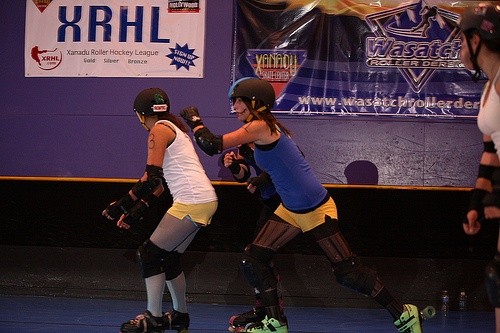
[222,77,286,333]
[180,76,423,333]
[103,87,219,333]
[457,5,500,333]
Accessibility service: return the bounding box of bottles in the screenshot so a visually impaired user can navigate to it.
[440,290,448,311]
[459,292,467,311]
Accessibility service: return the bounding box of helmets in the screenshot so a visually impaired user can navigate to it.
[228,77,276,108]
[461,3,500,46]
[134,88,170,115]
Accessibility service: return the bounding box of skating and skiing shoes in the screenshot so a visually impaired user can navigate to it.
[120,310,169,333]
[228,305,285,332]
[162,307,190,333]
[245,315,288,333]
[394,304,435,333]
